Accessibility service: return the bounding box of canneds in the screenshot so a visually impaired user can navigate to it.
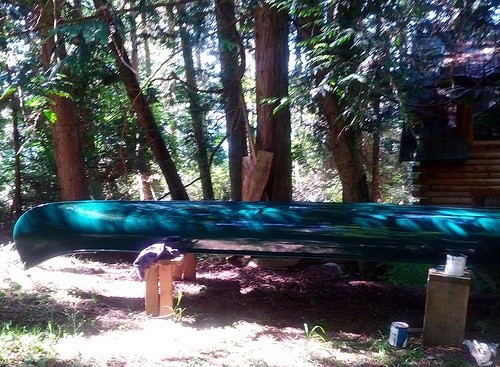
[388,321,410,347]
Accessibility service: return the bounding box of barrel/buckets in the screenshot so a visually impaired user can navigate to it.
[443,253,468,276]
[388,322,410,347]
